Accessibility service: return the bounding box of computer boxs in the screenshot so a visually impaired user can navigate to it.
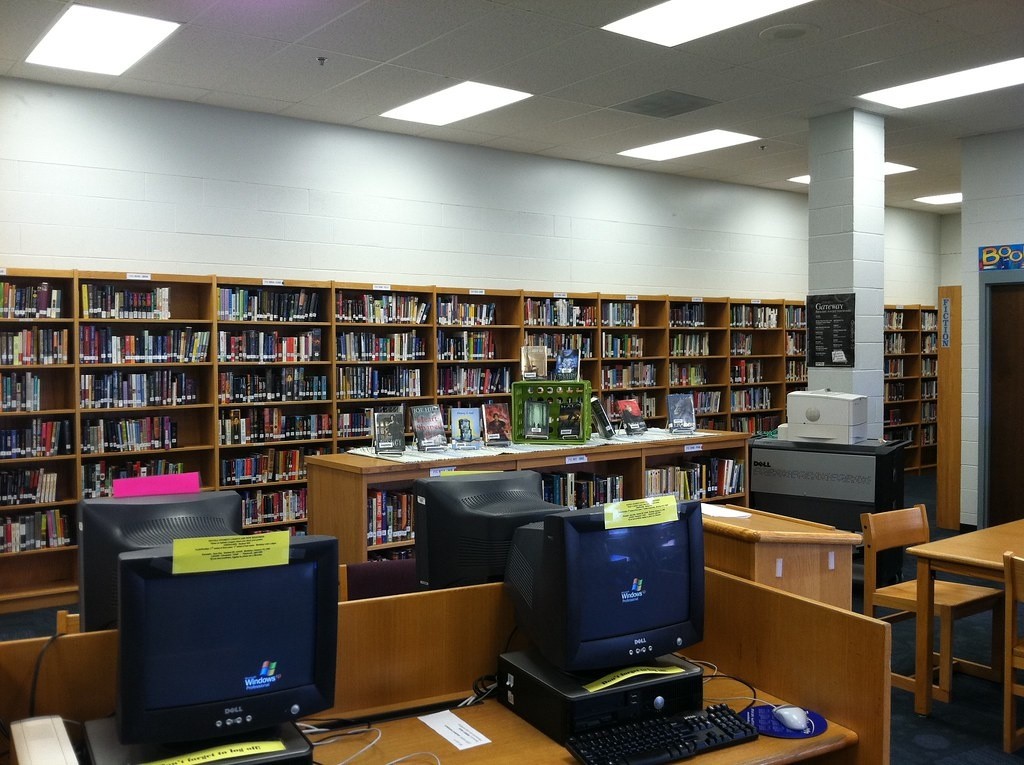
[498,650,703,748]
[86,716,314,765]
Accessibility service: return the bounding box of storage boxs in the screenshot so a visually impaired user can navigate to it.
[511,381,593,444]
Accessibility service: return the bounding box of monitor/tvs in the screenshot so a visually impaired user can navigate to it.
[507,496,704,679]
[79,490,242,634]
[412,469,570,592]
[116,533,338,748]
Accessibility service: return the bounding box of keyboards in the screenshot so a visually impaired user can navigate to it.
[565,703,760,765]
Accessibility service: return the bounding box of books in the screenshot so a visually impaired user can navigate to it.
[615,399,647,436]
[366,484,415,563]
[669,301,726,431]
[79,279,212,500]
[373,411,407,453]
[539,470,624,510]
[920,310,938,444]
[437,294,509,394]
[601,300,657,418]
[453,407,482,444]
[784,305,808,381]
[525,296,597,382]
[591,396,616,438]
[335,290,432,437]
[216,286,332,540]
[646,457,746,502]
[730,305,782,434]
[410,404,447,451]
[0,282,76,554]
[884,310,914,446]
[484,402,514,444]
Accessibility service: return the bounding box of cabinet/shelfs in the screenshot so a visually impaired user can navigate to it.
[0,265,938,616]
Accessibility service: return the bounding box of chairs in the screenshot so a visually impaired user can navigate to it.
[859,502,1024,753]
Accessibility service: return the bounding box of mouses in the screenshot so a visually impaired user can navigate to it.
[772,705,808,730]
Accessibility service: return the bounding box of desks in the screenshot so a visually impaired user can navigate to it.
[1,566,892,765]
[905,518,1024,716]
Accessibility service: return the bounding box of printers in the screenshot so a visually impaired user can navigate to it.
[786,387,868,444]
